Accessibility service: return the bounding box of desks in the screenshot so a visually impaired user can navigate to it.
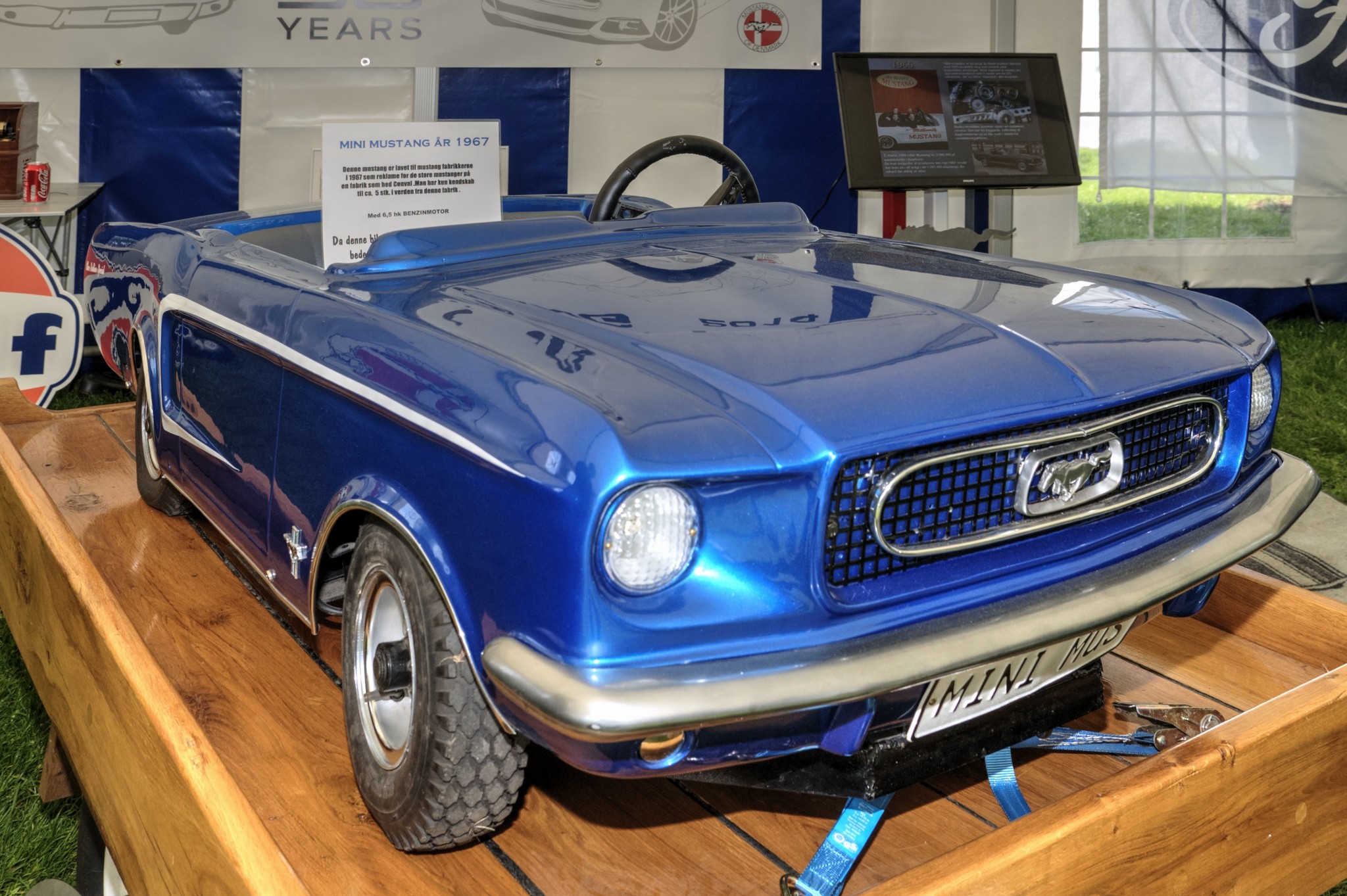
[0,182,105,291]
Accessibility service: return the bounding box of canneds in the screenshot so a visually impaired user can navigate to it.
[22,161,51,203]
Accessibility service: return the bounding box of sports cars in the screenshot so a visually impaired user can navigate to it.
[85,137,1321,852]
